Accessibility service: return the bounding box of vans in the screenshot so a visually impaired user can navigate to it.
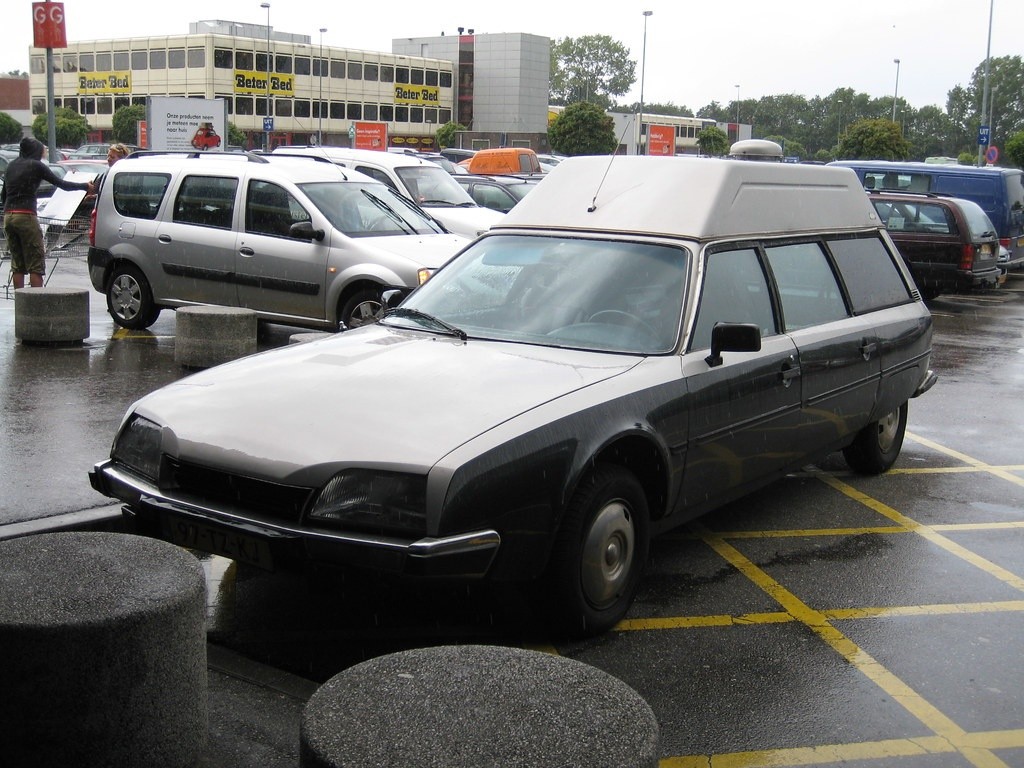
[86,149,519,335]
[276,143,510,240]
[818,159,1024,271]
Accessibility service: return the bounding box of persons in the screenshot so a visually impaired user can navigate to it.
[83,144,130,210]
[0,137,94,292]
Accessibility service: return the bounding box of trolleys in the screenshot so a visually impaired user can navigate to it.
[0,211,90,300]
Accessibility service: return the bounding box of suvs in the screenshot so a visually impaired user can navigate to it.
[864,189,1003,308]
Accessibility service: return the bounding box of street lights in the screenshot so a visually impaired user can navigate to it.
[902,109,910,137]
[836,100,843,149]
[735,84,741,125]
[262,3,271,151]
[637,10,654,154]
[318,27,328,144]
[893,58,901,124]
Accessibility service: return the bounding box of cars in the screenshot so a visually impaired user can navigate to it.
[0,144,125,217]
[88,140,937,640]
[373,139,567,215]
